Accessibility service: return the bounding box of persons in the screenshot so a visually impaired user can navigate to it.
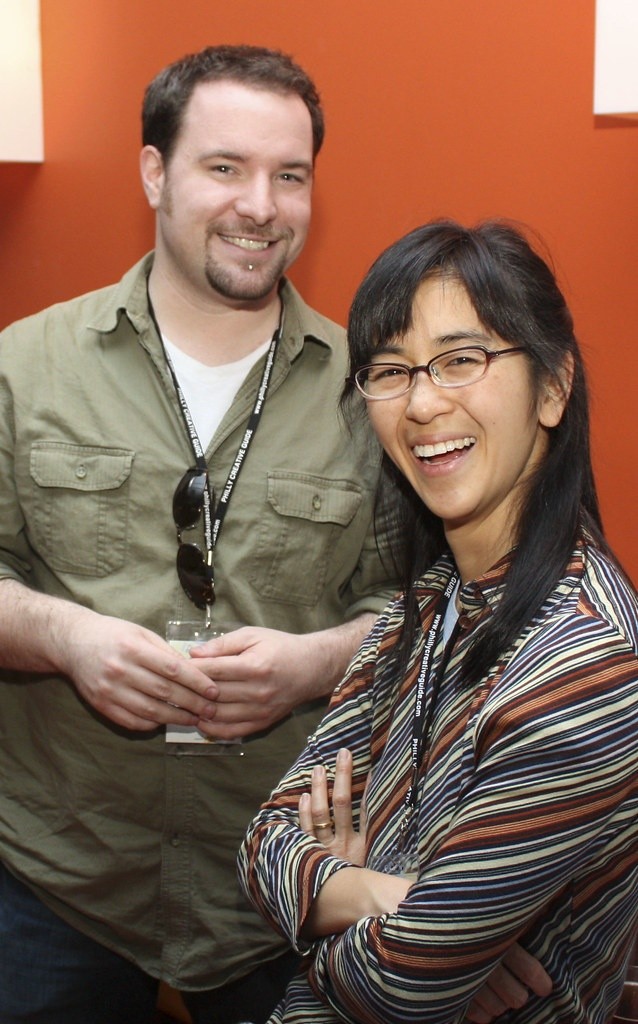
[0,46,423,1024]
[238,213,638,1024]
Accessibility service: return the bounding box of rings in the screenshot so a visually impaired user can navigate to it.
[313,822,333,830]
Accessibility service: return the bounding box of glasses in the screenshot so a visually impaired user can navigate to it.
[341,345,529,401]
[171,466,215,610]
